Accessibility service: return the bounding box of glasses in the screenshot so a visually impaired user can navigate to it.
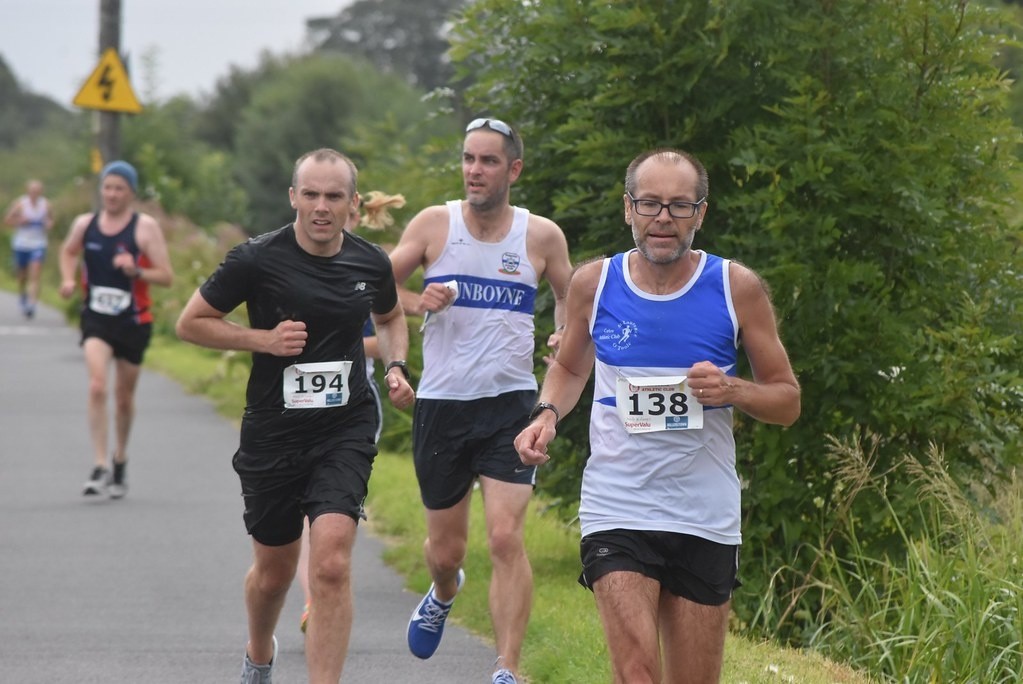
[627,191,705,219]
[466,117,520,158]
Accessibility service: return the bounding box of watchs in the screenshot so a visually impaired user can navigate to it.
[385,360,410,380]
[528,402,559,421]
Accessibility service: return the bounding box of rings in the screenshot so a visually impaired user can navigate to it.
[700,389,703,397]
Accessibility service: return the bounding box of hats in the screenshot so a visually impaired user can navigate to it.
[103,159,137,195]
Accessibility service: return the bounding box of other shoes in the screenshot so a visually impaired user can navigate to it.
[302,599,311,633]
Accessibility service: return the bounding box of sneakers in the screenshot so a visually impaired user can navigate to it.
[240,634,279,684]
[405,567,465,659]
[492,670,517,684]
[108,458,127,499]
[82,468,107,495]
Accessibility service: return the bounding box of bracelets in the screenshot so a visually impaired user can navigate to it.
[559,325,565,330]
[137,268,141,279]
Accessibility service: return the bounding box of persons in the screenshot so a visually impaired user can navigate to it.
[388,119,573,684]
[60,160,174,498]
[175,148,416,684]
[4,181,54,318]
[514,148,801,684]
[300,213,380,631]
